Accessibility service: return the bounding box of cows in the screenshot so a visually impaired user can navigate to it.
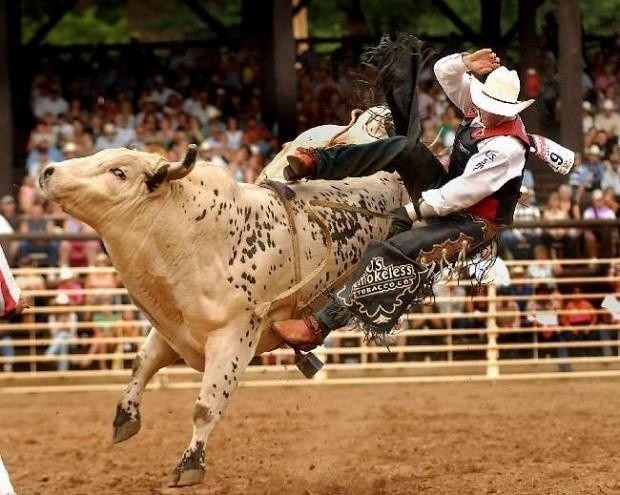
[36,104,429,488]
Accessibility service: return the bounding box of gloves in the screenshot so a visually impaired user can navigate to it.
[384,206,414,242]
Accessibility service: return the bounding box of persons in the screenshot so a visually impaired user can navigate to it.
[270,47,575,350]
[368,9,620,369]
[0,28,373,370]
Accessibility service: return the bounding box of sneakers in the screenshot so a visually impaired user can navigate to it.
[282,152,315,182]
[271,313,331,352]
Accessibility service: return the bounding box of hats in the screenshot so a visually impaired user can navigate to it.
[469,66,536,117]
